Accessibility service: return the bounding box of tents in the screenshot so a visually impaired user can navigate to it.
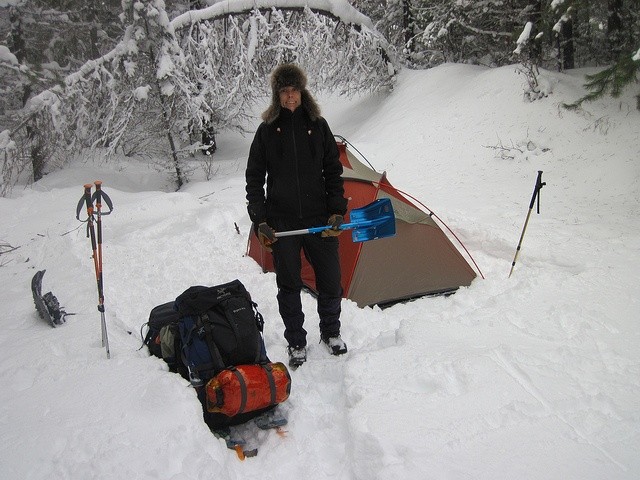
[245,133,485,309]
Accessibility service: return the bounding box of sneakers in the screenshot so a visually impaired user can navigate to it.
[319,334,347,356]
[287,343,307,368]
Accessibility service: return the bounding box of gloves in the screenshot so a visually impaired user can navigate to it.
[321,214,344,238]
[257,222,278,253]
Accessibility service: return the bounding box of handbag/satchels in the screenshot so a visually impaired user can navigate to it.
[135,300,179,359]
[205,362,291,418]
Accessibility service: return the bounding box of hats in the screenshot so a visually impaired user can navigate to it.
[261,63,323,125]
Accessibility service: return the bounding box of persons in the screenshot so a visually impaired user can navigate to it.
[246,64,348,368]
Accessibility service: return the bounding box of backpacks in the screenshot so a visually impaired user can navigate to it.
[168,279,280,431]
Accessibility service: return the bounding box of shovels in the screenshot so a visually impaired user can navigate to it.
[273,198,395,243]
